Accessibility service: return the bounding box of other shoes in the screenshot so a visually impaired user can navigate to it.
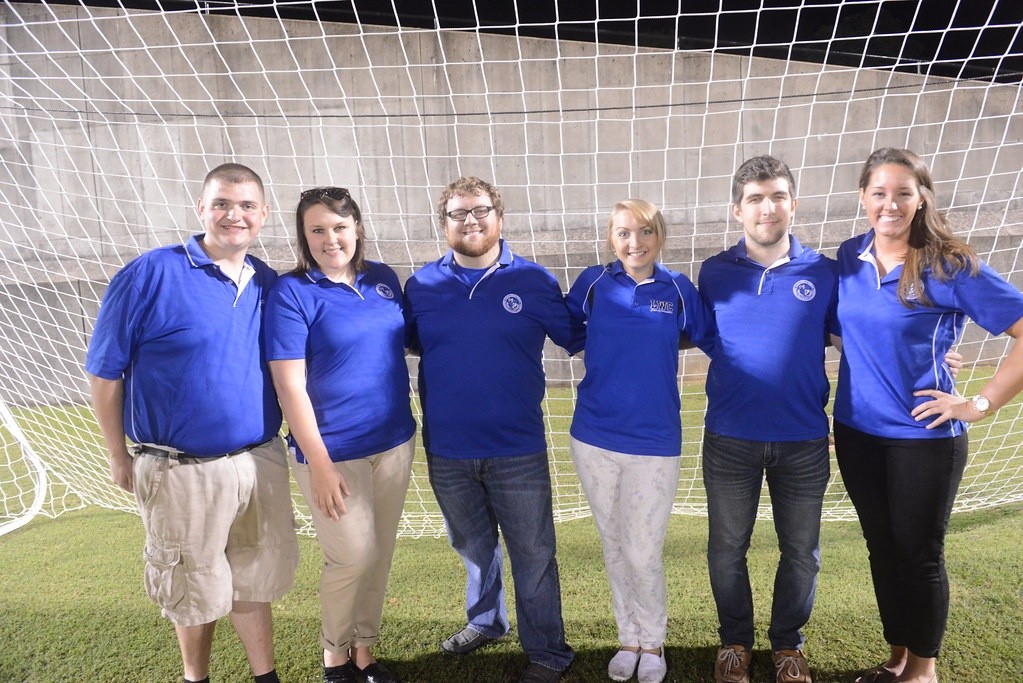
[349,656,400,683]
[637,643,667,683]
[855,660,940,683]
[518,661,561,683]
[440,627,490,654]
[607,645,643,682]
[320,658,356,683]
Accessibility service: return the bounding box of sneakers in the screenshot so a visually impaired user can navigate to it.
[771,646,812,683]
[713,639,751,683]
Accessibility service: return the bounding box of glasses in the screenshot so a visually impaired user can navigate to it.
[446,205,495,222]
[301,187,353,209]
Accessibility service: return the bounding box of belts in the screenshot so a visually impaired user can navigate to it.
[142,437,275,460]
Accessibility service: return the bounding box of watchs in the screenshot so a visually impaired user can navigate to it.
[972,394,996,416]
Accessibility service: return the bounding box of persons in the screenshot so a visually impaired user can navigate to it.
[828,147,1023,683]
[264,186,420,683]
[85,162,298,683]
[679,154,964,683]
[403,175,587,683]
[565,197,718,683]
